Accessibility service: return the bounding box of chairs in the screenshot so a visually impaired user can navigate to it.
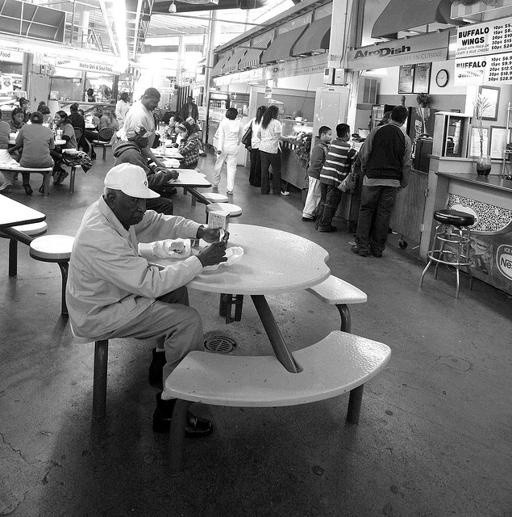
[92,127,114,160]
[73,127,83,151]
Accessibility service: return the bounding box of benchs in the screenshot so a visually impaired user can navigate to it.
[220,273,367,333]
[69,315,108,425]
[0,158,81,199]
[162,330,392,423]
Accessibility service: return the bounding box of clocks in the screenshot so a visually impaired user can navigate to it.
[436,68,448,87]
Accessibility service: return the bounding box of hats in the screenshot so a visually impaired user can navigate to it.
[30,112,43,123]
[104,162,161,199]
[125,124,154,139]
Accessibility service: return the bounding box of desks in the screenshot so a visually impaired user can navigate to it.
[148,147,212,195]
[138,223,331,373]
[8,140,67,146]
[0,194,48,239]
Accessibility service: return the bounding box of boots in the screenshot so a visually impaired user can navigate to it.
[153,392,213,438]
[149,347,167,389]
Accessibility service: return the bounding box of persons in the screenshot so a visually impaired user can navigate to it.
[259,105,295,195]
[313,122,360,233]
[210,107,241,196]
[299,125,332,220]
[114,124,179,216]
[243,105,268,189]
[64,161,231,437]
[123,87,167,169]
[153,94,201,170]
[0,86,113,197]
[346,109,394,237]
[114,92,131,141]
[351,104,413,258]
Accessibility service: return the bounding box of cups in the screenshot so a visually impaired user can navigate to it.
[57,130,64,139]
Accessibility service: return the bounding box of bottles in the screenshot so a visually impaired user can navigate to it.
[52,128,56,140]
[159,140,166,155]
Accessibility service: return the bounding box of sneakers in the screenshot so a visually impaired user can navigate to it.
[351,245,368,256]
[53,170,69,186]
[39,186,50,195]
[25,184,33,194]
[315,222,337,232]
[302,216,317,222]
[214,184,233,195]
[370,247,382,257]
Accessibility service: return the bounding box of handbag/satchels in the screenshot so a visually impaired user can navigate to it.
[241,127,252,148]
[338,164,362,196]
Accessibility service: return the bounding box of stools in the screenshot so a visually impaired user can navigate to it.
[192,173,242,224]
[9,220,75,318]
[419,209,474,299]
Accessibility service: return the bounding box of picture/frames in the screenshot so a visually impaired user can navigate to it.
[398,62,431,94]
